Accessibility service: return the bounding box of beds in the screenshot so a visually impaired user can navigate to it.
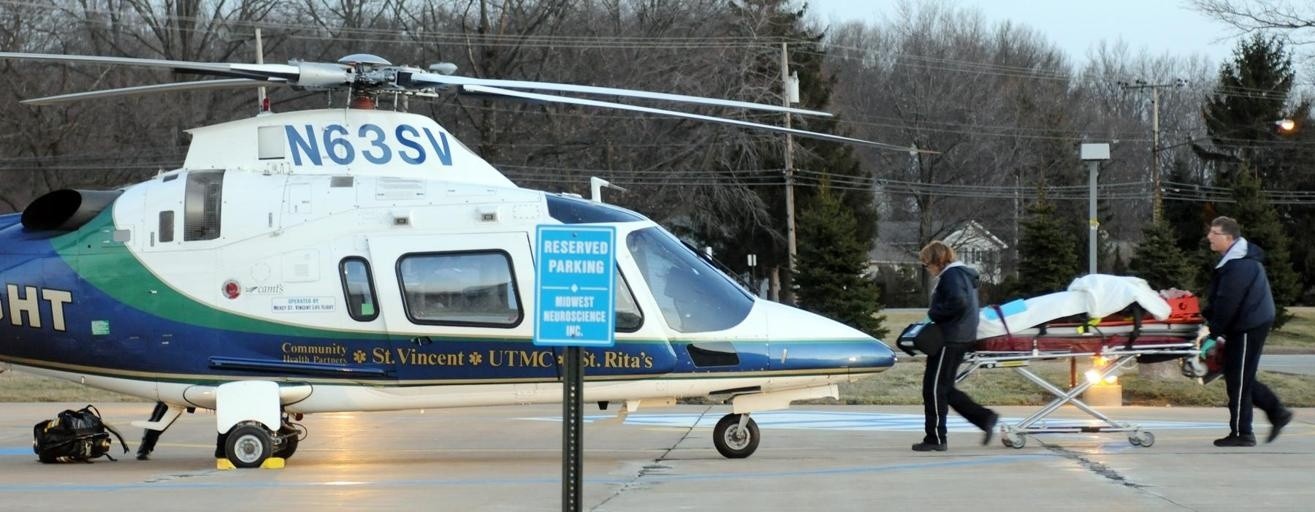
[894,320,1221,450]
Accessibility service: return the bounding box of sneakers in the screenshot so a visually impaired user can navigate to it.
[1214,435,1256,446]
[984,413,997,445]
[912,442,947,452]
[1268,414,1292,443]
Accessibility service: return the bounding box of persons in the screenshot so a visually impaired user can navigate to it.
[1023,284,1197,330]
[907,238,1001,452]
[1200,214,1294,447]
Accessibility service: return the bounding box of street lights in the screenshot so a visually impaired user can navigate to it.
[1080,141,1112,275]
[1150,119,1294,224]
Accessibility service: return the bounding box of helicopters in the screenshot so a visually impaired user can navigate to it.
[0,53,944,469]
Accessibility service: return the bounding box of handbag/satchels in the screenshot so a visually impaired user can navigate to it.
[32,403,110,463]
[896,323,942,358]
